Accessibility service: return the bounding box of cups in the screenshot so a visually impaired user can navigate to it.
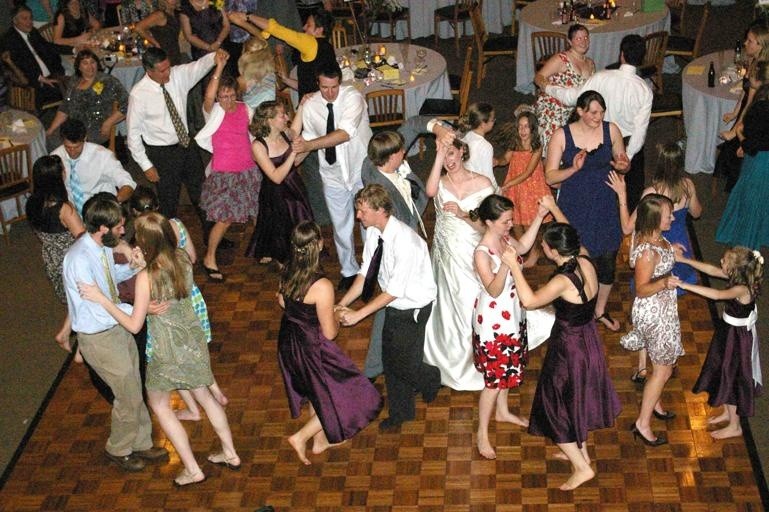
[417,47,427,64]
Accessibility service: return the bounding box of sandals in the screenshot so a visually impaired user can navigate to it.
[596,312,620,331]
[208,452,241,469]
[172,470,207,486]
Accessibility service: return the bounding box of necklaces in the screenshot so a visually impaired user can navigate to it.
[447,170,474,200]
[192,0,207,9]
[567,49,586,62]
[654,234,664,242]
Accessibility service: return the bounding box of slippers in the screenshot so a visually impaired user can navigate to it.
[202,261,224,283]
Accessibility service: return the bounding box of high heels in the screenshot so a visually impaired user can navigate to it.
[632,368,647,385]
[653,408,676,420]
[632,423,668,447]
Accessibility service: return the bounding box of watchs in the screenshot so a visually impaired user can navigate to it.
[245,11,254,22]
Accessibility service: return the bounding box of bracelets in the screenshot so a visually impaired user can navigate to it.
[210,75,222,80]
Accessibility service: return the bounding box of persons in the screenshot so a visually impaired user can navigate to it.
[460,101,504,196]
[194,53,273,283]
[422,136,555,393]
[24,154,87,364]
[26,0,58,30]
[500,194,623,491]
[6,4,88,113]
[125,47,236,249]
[359,114,455,384]
[292,62,374,290]
[603,141,704,384]
[226,13,277,114]
[226,9,343,109]
[670,245,765,439]
[133,0,183,67]
[714,23,769,194]
[532,23,597,158]
[45,48,130,153]
[543,90,632,333]
[468,193,551,460]
[61,198,169,472]
[76,211,242,488]
[79,191,147,407]
[543,33,654,215]
[333,182,442,432]
[243,100,316,272]
[494,110,554,268]
[179,0,230,104]
[49,118,138,220]
[54,0,100,46]
[274,219,384,467]
[130,185,229,423]
[714,59,768,251]
[619,192,688,446]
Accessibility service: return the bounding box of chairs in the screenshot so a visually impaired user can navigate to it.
[531,31,571,101]
[665,2,710,62]
[9,81,35,115]
[511,0,534,38]
[365,89,407,132]
[419,48,471,160]
[639,31,668,96]
[469,1,517,89]
[274,92,296,121]
[0,144,35,247]
[434,0,482,60]
[36,25,59,44]
[330,26,348,50]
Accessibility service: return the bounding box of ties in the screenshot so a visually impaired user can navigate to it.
[361,237,384,304]
[67,158,85,223]
[399,174,429,239]
[161,83,191,148]
[325,105,337,167]
[101,248,123,305]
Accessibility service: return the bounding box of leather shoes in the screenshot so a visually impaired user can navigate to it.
[104,450,145,469]
[136,444,167,458]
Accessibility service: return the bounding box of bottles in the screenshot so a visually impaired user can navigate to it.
[707,61,716,88]
[734,38,742,65]
[605,0,611,20]
[561,1,569,24]
[570,0,576,21]
